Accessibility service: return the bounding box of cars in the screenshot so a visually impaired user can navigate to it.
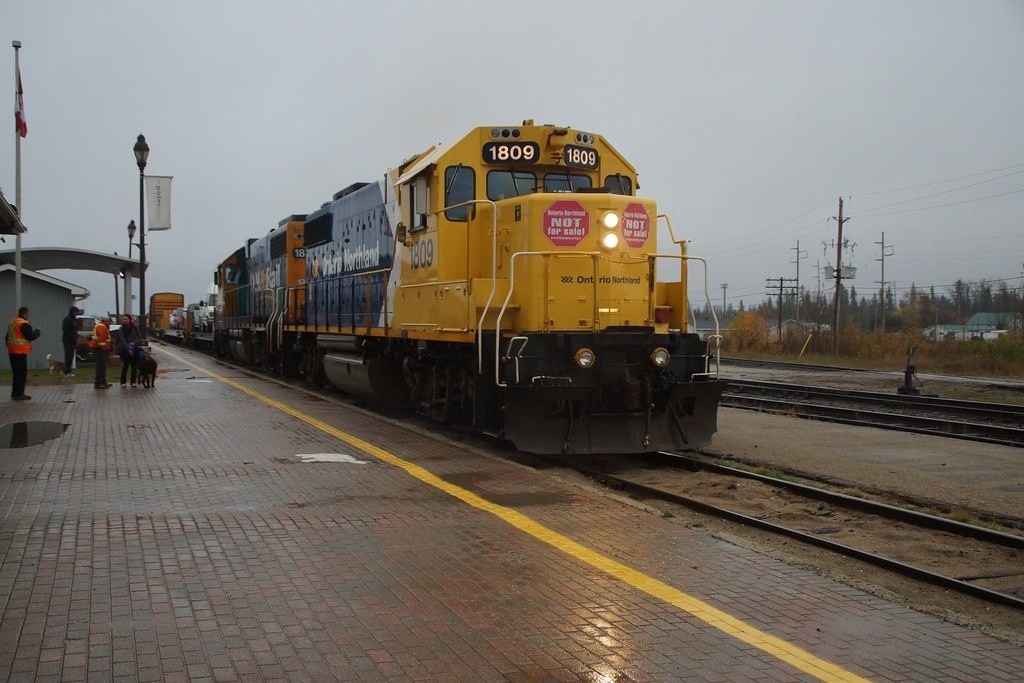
[169,293,217,332]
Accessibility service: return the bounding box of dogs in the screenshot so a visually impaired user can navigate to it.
[136,353,158,388]
[46,352,65,375]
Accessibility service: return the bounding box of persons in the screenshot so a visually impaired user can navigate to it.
[89,316,113,389]
[62,306,81,377]
[115,314,141,388]
[5,306,40,400]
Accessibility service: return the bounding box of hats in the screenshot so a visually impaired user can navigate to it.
[102,315,113,323]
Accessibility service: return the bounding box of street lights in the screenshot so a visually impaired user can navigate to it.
[133,134,151,345]
[127,220,136,259]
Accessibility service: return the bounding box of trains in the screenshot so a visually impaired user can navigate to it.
[150,119,726,454]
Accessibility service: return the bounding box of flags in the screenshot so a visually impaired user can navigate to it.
[15,67,27,138]
[144,174,174,231]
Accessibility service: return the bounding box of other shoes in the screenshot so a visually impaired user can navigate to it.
[95,384,112,388]
[131,383,137,386]
[12,394,31,400]
[64,372,76,376]
[122,384,126,386]
